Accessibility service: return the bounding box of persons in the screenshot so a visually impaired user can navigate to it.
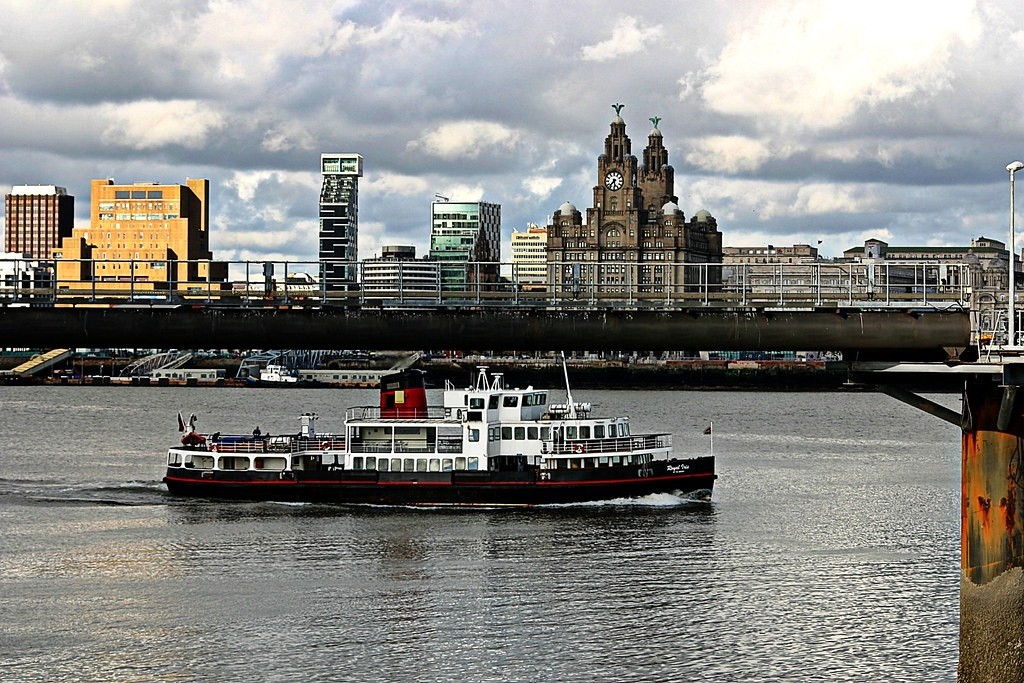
[264,432,270,436]
[253,426,261,436]
[212,432,220,443]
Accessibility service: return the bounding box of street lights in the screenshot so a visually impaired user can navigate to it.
[1006,159,1023,345]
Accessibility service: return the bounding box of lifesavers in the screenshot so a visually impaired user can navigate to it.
[321,441,331,451]
[575,443,585,453]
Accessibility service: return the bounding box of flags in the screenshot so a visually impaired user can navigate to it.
[818,241,822,244]
[178,414,184,432]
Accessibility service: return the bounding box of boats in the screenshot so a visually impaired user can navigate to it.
[164,353,719,509]
